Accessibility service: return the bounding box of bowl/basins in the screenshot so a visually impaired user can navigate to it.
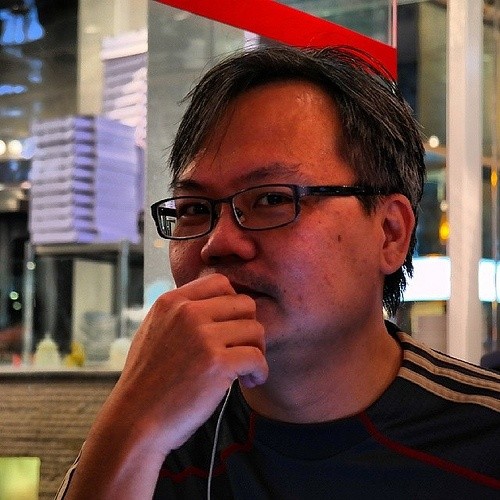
[80,310,117,360]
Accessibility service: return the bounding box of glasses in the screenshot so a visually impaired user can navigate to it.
[149,179,393,242]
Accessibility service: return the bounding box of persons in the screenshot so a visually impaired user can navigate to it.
[52,40,500,500]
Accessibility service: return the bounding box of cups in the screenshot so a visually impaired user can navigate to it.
[0,456,40,500]
